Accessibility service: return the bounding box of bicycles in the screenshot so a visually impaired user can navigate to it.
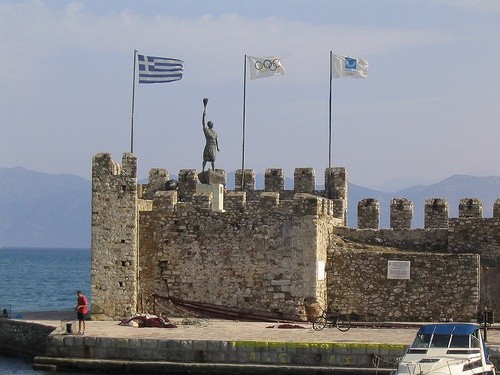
[312,304,352,333]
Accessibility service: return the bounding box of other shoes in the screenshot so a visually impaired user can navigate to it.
[77,331,86,335]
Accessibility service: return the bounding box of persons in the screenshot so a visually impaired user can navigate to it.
[202,111,219,172]
[75,291,88,335]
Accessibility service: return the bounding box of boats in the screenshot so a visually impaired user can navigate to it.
[394,324,499,375]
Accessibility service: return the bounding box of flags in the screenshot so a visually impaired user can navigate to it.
[247,55,287,80]
[137,55,184,85]
[332,53,368,79]
